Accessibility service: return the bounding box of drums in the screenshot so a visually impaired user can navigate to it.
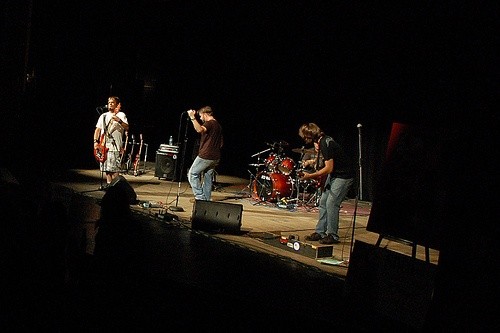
[277,157,296,175]
[263,151,280,170]
[253,171,296,203]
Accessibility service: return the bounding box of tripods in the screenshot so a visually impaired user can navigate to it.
[248,146,322,212]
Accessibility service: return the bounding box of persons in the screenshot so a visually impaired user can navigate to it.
[187,106,223,201]
[299,123,354,245]
[94,96,129,188]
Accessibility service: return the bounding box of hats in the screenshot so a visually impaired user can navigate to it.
[97,189,132,214]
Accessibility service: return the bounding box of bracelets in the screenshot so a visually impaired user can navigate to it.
[191,118,196,120]
[118,119,122,124]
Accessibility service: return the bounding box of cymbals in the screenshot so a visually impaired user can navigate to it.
[292,148,312,154]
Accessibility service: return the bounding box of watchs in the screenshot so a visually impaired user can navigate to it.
[94,139,98,143]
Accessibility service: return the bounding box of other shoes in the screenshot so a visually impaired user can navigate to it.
[304,232,323,241]
[319,234,341,244]
[100,183,110,190]
[190,199,195,203]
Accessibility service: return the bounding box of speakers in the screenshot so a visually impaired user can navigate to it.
[109,174,137,203]
[191,199,243,234]
[154,152,179,179]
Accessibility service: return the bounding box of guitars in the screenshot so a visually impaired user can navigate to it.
[134,133,145,176]
[121,129,130,167]
[93,102,122,163]
[125,134,136,173]
[297,170,329,190]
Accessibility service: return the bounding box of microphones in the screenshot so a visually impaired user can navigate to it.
[97,104,108,109]
[183,109,196,115]
